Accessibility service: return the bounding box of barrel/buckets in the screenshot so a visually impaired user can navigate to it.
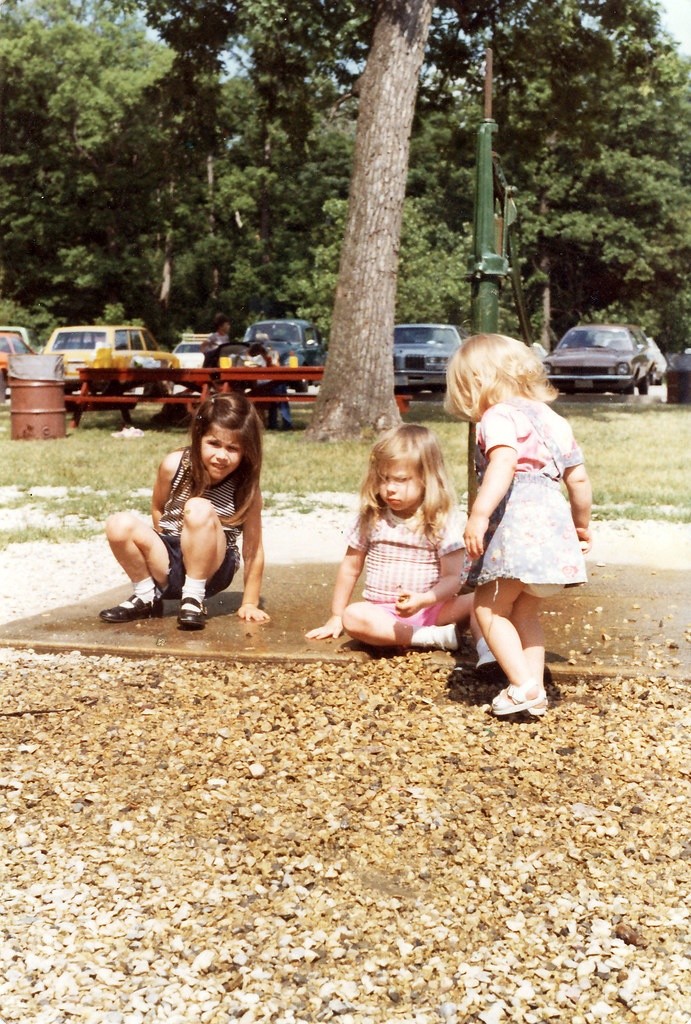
[9,354,67,440]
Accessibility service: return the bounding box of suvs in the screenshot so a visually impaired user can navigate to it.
[42,324,179,379]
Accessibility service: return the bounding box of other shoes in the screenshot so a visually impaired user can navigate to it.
[422,623,459,650]
[476,644,498,672]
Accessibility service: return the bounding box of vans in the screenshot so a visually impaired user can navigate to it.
[0,332,41,382]
[0,324,31,345]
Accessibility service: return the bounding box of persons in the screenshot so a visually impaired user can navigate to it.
[243,344,295,432]
[443,334,591,717]
[200,319,230,366]
[304,424,553,686]
[98,392,272,629]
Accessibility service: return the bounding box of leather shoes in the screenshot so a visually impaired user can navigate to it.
[178,597,208,630]
[99,595,164,622]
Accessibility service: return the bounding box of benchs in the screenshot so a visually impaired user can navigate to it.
[65,394,200,415]
[247,393,412,415]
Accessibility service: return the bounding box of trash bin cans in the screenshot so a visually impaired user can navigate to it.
[5,352,67,440]
[666,351,690,403]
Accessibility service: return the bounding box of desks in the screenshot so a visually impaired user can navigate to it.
[217,365,324,429]
[70,368,221,432]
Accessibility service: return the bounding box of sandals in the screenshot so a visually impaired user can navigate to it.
[491,678,549,716]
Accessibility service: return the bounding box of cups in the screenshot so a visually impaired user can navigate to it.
[220,357,232,368]
[290,357,298,368]
[87,347,131,368]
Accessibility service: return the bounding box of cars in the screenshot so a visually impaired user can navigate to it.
[240,320,327,368]
[171,333,211,369]
[394,324,463,394]
[543,323,667,396]
